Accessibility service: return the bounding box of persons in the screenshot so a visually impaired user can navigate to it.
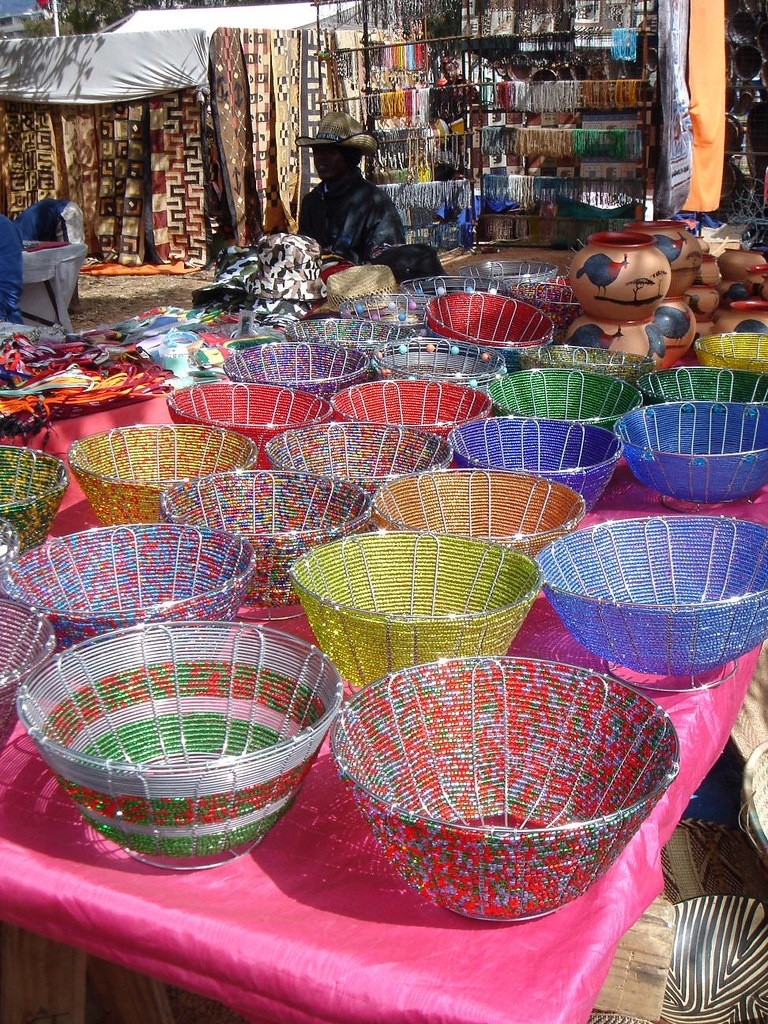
[0,198,84,325]
[295,113,407,266]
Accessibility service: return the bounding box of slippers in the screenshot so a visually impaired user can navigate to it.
[734,82,754,116]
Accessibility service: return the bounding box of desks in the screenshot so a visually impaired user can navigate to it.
[0,301,768,1024]
[19,240,87,333]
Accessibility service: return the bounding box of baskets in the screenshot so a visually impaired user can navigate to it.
[166,381,333,470]
[613,401,768,516]
[331,378,492,440]
[0,445,69,561]
[289,531,544,693]
[537,516,768,692]
[446,415,625,516]
[635,365,768,404]
[371,467,586,560]
[0,522,255,655]
[223,261,768,382]
[330,656,681,922]
[486,368,643,431]
[0,596,57,746]
[265,421,453,500]
[16,621,343,871]
[159,469,373,621]
[69,423,259,524]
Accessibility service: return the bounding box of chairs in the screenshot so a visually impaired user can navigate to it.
[15,198,85,244]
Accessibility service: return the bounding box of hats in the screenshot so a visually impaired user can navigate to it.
[192,245,249,311]
[245,233,326,327]
[295,112,378,155]
[371,243,453,295]
[314,264,401,313]
